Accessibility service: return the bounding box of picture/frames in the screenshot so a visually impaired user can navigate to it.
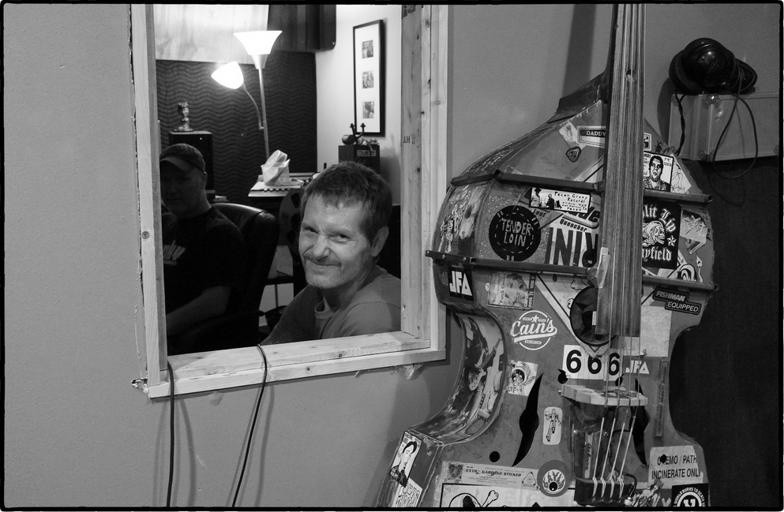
[351,18,387,138]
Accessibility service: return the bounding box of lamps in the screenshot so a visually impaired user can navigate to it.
[208,29,286,161]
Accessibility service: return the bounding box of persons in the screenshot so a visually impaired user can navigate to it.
[643,156,671,191]
[159,143,249,356]
[437,218,455,253]
[389,441,417,487]
[260,160,401,346]
[530,188,554,209]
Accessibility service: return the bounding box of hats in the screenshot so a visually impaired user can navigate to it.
[160,143,205,174]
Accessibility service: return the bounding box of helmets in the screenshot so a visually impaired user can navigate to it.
[674,38,757,93]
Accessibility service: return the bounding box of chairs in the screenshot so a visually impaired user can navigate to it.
[182,192,311,350]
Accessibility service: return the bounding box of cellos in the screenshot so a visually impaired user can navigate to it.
[377,2,716,511]
